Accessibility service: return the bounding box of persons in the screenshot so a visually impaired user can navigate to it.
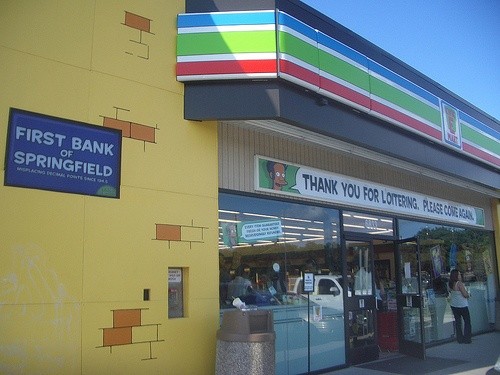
[225,268,253,297]
[446,267,473,345]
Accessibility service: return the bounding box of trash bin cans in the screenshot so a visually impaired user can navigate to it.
[214,309,276,375]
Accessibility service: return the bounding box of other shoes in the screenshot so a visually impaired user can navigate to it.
[457,336,472,344]
